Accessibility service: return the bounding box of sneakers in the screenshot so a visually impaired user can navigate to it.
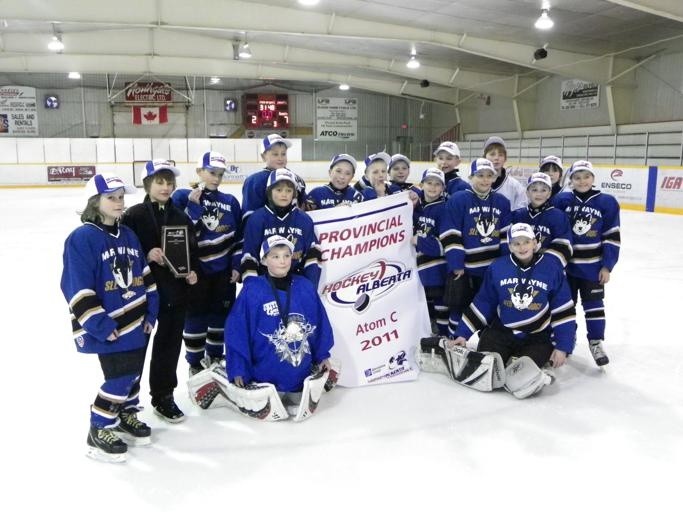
[87,416,127,453]
[153,397,184,423]
[114,405,151,437]
[589,340,609,366]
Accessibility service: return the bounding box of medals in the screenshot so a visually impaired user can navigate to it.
[198,182,206,192]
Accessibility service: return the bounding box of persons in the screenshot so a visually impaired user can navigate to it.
[60,171,162,457]
[172,149,245,381]
[237,132,622,371]
[111,156,202,433]
[221,234,335,390]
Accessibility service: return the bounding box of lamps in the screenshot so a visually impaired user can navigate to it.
[406,56,419,69]
[239,42,251,58]
[47,34,63,51]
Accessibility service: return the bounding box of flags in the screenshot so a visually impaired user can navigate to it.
[131,103,169,125]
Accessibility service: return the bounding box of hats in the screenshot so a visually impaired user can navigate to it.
[421,136,595,189]
[330,152,411,170]
[86,135,297,200]
[507,223,535,244]
[259,234,295,260]
